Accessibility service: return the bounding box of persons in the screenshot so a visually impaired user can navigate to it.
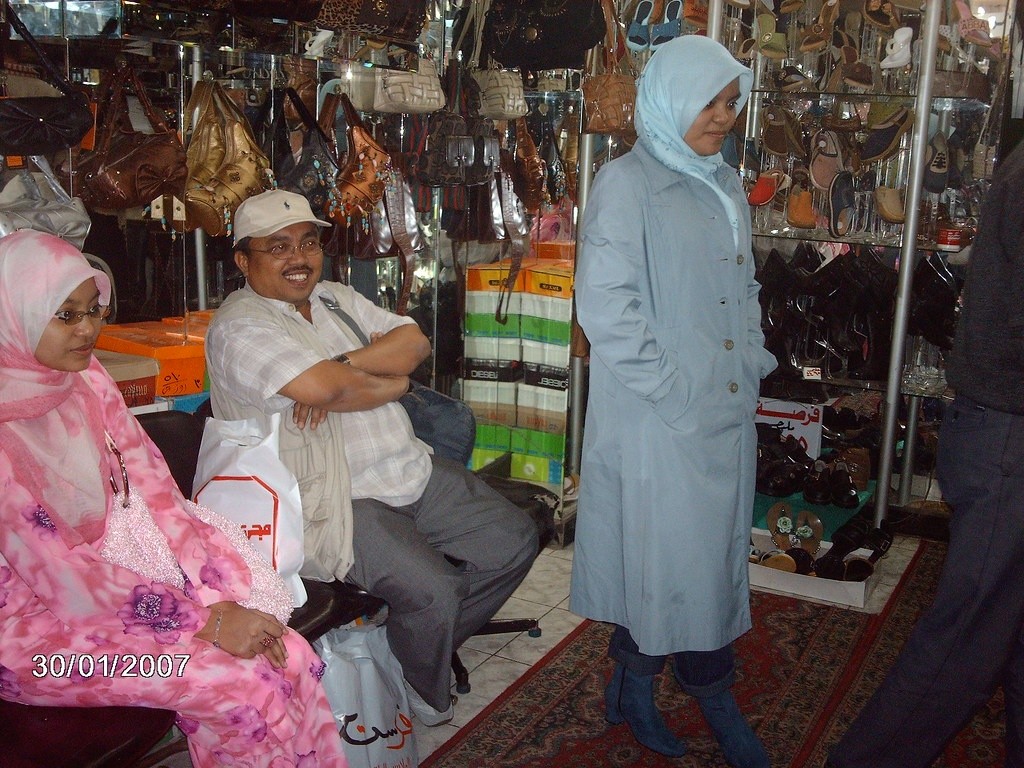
[826,141,1024,768]
[0,228,348,768]
[569,35,779,768]
[206,189,540,728]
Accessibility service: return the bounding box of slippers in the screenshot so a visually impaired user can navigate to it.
[685,0,709,27]
[753,502,893,582]
[626,0,654,52]
[649,0,683,51]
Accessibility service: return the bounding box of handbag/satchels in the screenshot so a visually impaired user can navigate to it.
[191,413,309,608]
[313,625,419,768]
[73,0,637,260]
[0,0,94,154]
[973,143,996,181]
[399,379,475,467]
[101,486,294,628]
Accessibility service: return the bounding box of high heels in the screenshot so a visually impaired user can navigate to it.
[696,690,772,768]
[604,662,687,758]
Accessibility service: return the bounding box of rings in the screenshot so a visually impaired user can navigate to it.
[262,635,273,647]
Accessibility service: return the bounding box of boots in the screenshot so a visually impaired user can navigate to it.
[798,260,863,356]
[906,252,966,350]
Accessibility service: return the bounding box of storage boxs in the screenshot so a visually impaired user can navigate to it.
[87,309,216,407]
[459,242,574,520]
[746,523,882,609]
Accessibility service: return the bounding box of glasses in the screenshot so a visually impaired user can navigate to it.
[52,303,112,325]
[248,241,325,260]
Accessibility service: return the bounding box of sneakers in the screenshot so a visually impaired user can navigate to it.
[403,678,453,726]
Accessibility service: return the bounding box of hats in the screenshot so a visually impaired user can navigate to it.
[232,189,332,248]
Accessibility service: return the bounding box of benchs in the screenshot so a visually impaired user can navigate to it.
[0,399,556,768]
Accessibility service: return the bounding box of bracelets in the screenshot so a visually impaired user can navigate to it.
[331,354,350,367]
[212,608,222,647]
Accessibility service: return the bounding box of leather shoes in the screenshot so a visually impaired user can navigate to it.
[830,463,859,508]
[804,460,832,504]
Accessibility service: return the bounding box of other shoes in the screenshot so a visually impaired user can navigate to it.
[724,0,993,238]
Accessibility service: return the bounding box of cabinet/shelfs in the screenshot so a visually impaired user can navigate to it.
[708,1,1014,536]
